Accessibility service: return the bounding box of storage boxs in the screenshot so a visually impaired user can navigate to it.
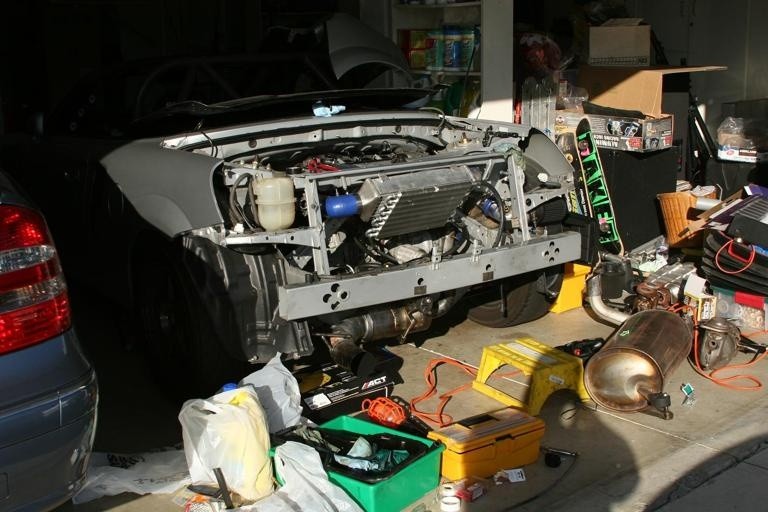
[292,362,397,427]
[269,414,445,511]
[426,409,546,482]
[554,108,673,154]
[577,25,652,67]
[717,132,768,164]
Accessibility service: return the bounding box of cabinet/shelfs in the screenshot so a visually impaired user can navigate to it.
[390,0,515,124]
[573,64,728,252]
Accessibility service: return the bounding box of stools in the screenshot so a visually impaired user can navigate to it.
[472,337,592,414]
